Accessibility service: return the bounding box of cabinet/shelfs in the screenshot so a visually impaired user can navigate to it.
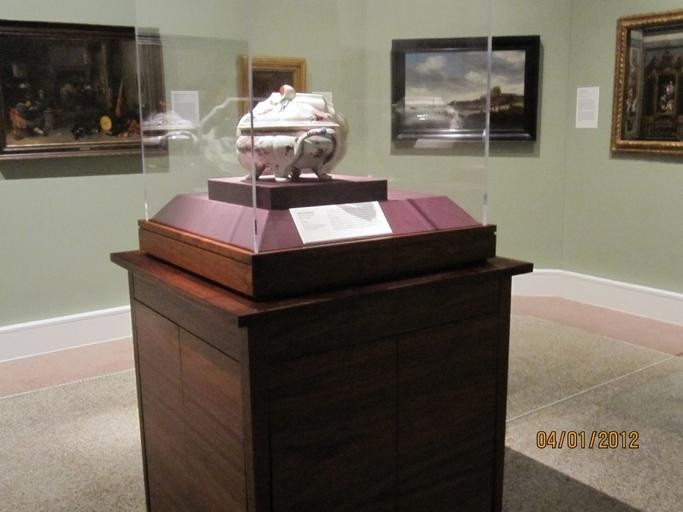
[111,251,533,512]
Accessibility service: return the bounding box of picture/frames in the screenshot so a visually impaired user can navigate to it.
[610,10,683,156]
[238,55,307,120]
[0,19,168,160]
[391,35,540,144]
